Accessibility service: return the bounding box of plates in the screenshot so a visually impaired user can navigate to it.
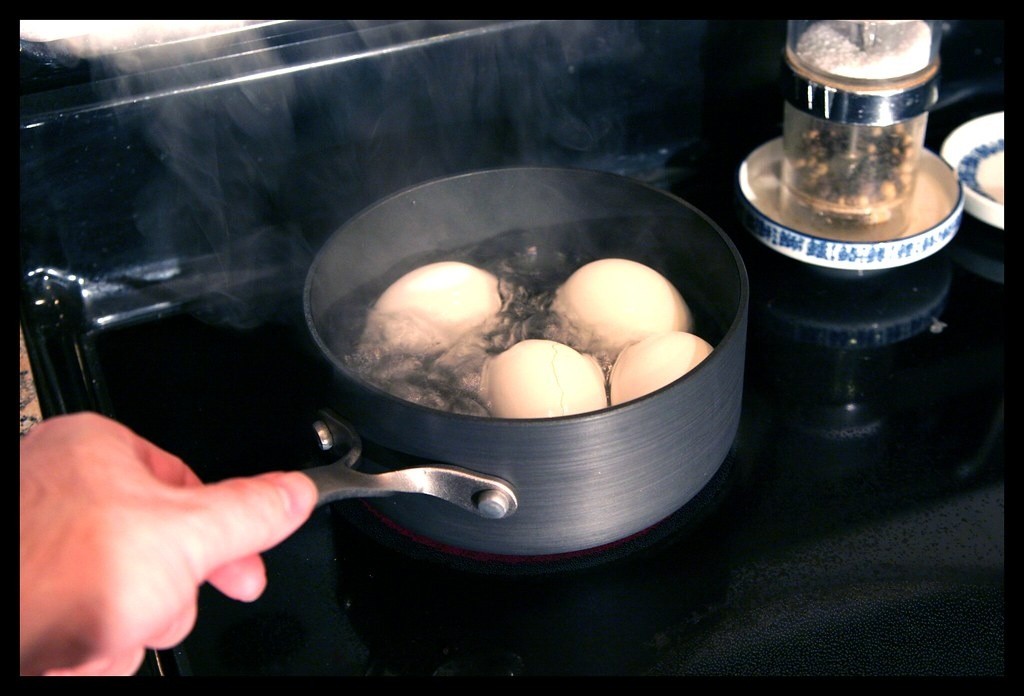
[939,104,1004,234]
[736,136,968,273]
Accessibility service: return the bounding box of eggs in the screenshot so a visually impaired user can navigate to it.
[367,258,715,419]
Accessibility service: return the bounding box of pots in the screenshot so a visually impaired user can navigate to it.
[301,160,752,561]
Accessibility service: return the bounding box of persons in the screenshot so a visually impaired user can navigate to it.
[21,412,318,675]
